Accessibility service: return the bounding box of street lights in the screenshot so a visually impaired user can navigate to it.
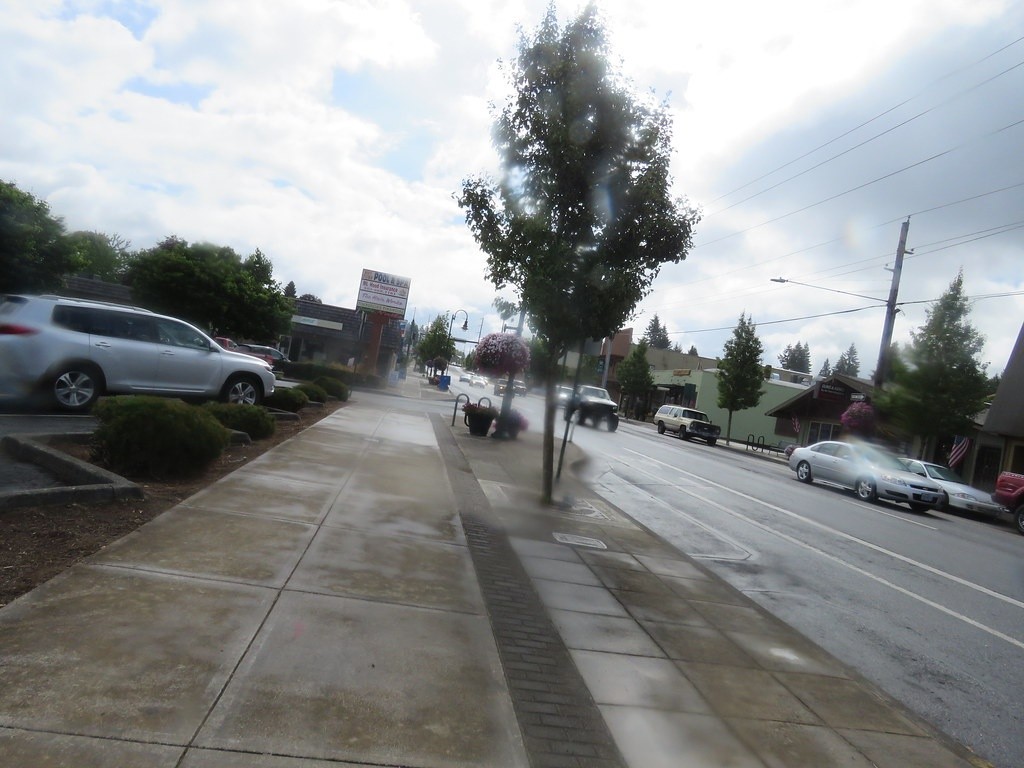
[442,310,468,377]
[771,277,899,408]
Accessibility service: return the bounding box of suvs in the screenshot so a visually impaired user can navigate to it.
[564,383,619,431]
[653,404,720,446]
[1,294,276,407]
[493,378,516,400]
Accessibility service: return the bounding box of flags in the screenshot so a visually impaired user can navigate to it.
[948,434,972,472]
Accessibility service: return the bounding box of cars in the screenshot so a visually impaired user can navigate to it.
[895,456,1003,521]
[459,371,489,387]
[452,362,462,368]
[555,386,576,407]
[513,381,528,397]
[789,440,945,512]
[215,336,275,370]
[240,343,293,370]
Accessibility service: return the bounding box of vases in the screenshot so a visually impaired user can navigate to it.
[504,428,518,438]
[469,414,494,436]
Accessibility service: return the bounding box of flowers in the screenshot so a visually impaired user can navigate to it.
[784,444,801,456]
[462,403,499,418]
[842,402,875,432]
[475,333,528,369]
[492,408,528,431]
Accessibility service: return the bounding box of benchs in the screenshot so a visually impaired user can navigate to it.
[768,441,795,456]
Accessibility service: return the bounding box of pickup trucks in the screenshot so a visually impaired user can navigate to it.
[994,470,1024,534]
